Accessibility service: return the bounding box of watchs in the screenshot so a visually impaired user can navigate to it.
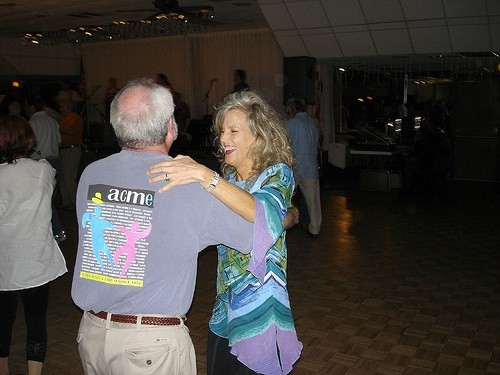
[204,170,219,193]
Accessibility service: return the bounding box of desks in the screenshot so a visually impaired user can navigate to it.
[349,146,400,171]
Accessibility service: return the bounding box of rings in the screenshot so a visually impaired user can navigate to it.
[165,174,169,182]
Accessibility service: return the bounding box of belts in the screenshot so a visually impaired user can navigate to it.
[88,310,180,325]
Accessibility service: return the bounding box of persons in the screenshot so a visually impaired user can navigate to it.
[284,96,322,239]
[0,88,84,246]
[146,90,303,375]
[104,77,121,148]
[233,69,249,93]
[156,74,190,146]
[71,77,299,375]
[0,114,68,375]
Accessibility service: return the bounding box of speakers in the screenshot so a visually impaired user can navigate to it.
[283,55,317,105]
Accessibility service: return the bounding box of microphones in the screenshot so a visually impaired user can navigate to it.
[209,79,216,83]
